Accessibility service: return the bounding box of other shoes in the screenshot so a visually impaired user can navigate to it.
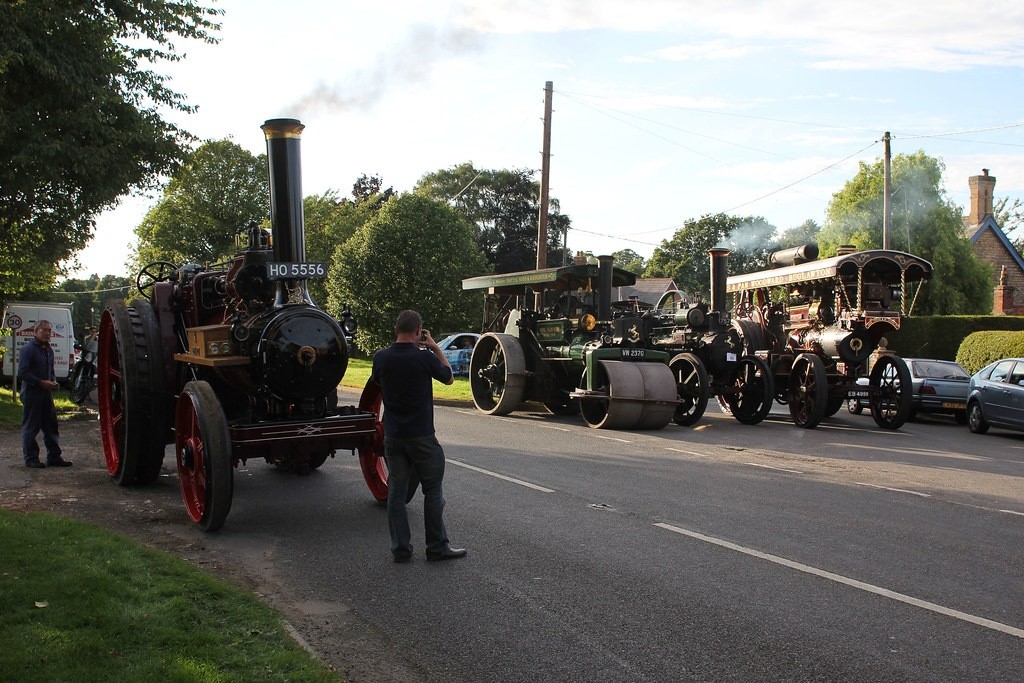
[46,455,73,467]
[26,458,46,468]
[427,546,467,561]
[394,544,413,563]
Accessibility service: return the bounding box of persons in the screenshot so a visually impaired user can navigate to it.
[371,310,467,563]
[19,320,73,468]
[84,327,99,363]
[461,338,472,348]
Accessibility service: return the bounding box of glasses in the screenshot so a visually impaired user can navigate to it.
[92,331,96,332]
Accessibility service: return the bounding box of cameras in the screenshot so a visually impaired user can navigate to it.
[419,331,426,341]
